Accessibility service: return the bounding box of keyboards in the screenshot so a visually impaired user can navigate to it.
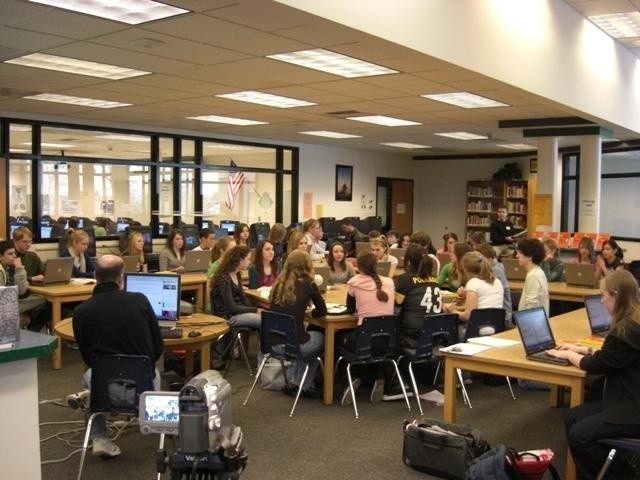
[160,328,183,338]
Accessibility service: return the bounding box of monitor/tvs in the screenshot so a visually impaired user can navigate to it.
[246,223,271,249]
[321,217,382,240]
[285,223,302,242]
[8,218,240,240]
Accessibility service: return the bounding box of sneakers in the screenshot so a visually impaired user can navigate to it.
[368,374,384,403]
[66,390,90,409]
[282,382,323,399]
[92,438,121,457]
[341,375,361,407]
[438,371,472,389]
[382,383,413,401]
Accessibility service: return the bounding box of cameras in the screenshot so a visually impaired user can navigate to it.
[139,369,231,455]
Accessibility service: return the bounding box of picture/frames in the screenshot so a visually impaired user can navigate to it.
[335,164,354,201]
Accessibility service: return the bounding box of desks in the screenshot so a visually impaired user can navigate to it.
[440,280,614,479]
[26,269,230,390]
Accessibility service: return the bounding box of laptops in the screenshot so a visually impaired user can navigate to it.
[501,256,527,282]
[355,242,409,276]
[512,306,585,366]
[180,250,209,274]
[123,272,181,327]
[121,256,140,274]
[563,262,600,289]
[437,252,452,276]
[311,266,330,294]
[32,257,74,286]
[583,293,612,337]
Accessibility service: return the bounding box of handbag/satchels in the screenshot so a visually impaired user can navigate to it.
[466,445,521,480]
[260,358,295,390]
[403,418,489,480]
[515,448,553,480]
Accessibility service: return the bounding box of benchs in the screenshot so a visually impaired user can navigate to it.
[29,238,167,264]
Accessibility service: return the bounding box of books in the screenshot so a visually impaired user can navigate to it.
[467,185,526,226]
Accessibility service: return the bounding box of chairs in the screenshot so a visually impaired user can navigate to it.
[77,352,179,479]
[596,435,639,479]
[218,267,517,418]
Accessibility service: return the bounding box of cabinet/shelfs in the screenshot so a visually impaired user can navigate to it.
[465,178,537,242]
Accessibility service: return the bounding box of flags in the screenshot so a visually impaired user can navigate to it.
[223,159,246,211]
